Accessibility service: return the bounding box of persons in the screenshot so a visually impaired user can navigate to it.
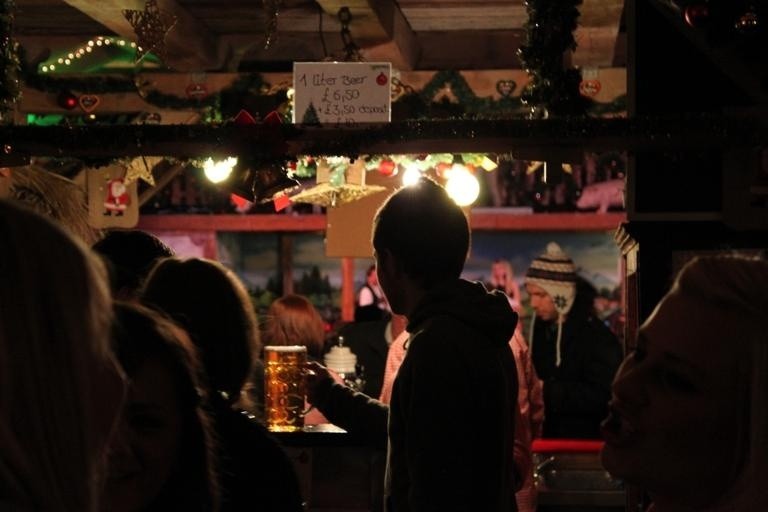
[0,161,768,511]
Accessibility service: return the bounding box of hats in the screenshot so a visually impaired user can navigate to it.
[525,241,578,367]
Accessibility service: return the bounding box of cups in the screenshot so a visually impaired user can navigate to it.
[265,343,317,434]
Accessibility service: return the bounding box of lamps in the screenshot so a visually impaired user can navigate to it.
[444,151,486,207]
